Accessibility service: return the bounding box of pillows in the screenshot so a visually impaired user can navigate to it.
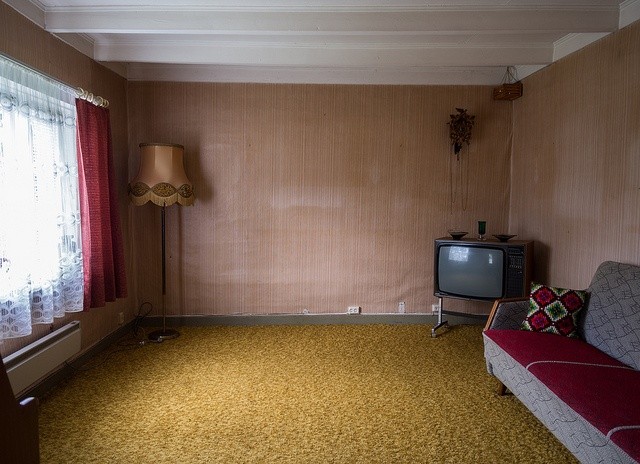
[518,280,589,341]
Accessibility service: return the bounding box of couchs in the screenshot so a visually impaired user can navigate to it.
[479,260,639,463]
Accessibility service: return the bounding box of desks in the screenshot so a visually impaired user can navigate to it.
[429,294,493,338]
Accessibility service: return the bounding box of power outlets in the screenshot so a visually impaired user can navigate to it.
[433,303,439,312]
[347,307,360,314]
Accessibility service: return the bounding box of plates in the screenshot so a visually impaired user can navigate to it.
[491,234,517,242]
[448,232,469,240]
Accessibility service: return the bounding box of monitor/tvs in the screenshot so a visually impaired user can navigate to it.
[432,236,534,305]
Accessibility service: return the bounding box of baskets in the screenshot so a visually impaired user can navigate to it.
[493,83,522,101]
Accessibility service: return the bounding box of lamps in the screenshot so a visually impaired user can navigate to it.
[127,139,196,344]
